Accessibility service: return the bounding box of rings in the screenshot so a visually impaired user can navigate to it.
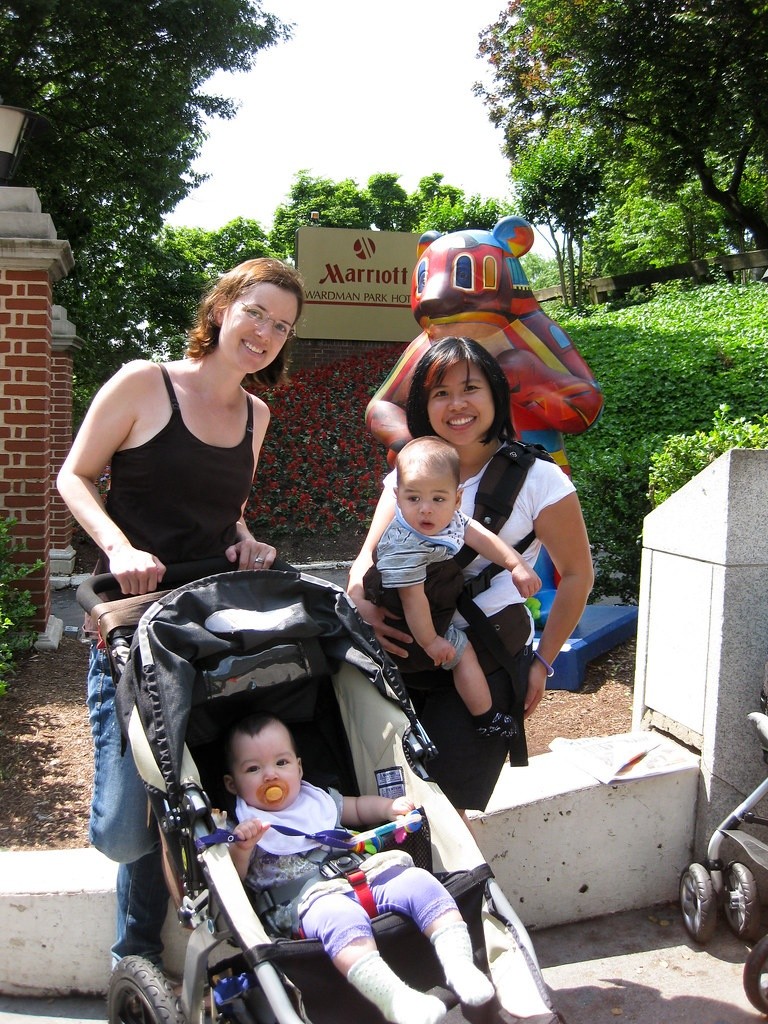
[255,559,264,563]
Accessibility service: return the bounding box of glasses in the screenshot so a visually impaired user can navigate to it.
[235,300,294,339]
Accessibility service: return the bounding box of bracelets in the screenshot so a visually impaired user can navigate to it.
[535,652,554,677]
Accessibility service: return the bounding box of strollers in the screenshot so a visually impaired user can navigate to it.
[78,554,562,1024]
[678,663,768,1015]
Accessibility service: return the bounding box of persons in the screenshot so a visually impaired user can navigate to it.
[56,259,304,969]
[224,714,496,1024]
[347,336,595,819]
[376,436,542,741]
